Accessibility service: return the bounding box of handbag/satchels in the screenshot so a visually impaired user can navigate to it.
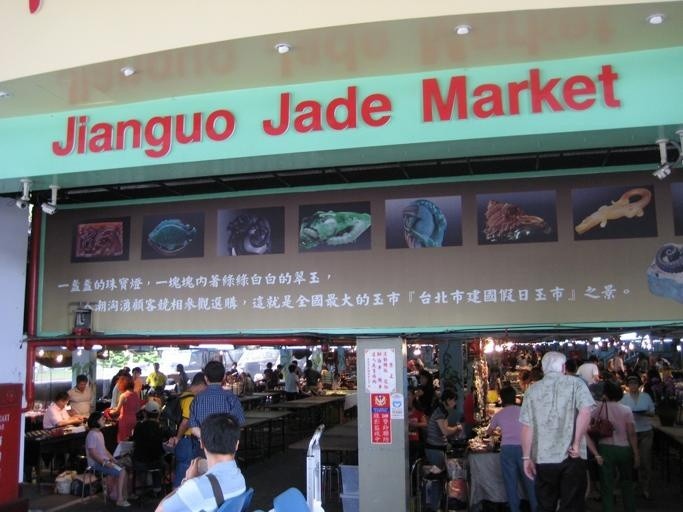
[71,473,98,497]
[55,470,77,494]
[587,421,613,439]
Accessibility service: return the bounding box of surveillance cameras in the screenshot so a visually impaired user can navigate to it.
[15,199,28,210]
[41,203,57,215]
[651,164,672,180]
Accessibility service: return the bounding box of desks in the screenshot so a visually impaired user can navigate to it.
[26,385,681,511]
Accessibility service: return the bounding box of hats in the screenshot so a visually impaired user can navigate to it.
[627,373,640,380]
[146,401,160,413]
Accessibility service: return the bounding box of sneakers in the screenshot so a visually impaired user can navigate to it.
[116,498,130,507]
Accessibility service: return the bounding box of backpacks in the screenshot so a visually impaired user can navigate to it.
[158,393,195,437]
